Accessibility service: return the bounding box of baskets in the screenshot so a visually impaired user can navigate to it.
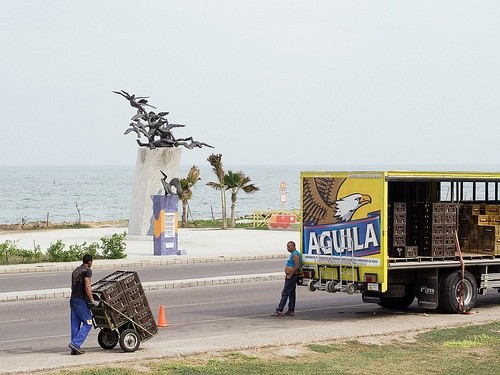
[388,202,456,258]
[88,268,157,342]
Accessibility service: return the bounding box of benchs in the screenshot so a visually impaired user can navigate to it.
[266,215,297,231]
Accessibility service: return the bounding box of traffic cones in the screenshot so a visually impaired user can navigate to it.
[157,305,169,327]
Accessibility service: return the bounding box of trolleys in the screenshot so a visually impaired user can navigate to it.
[86,293,154,353]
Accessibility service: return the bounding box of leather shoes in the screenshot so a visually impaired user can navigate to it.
[68,343,85,354]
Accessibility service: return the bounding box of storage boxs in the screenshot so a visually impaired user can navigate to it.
[90,270,159,341]
[388,202,500,256]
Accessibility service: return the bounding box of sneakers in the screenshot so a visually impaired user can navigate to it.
[271,311,283,317]
[283,311,294,316]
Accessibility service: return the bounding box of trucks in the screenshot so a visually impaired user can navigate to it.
[297,171,500,313]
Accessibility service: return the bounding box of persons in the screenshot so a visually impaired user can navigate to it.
[271,241,303,316]
[68,254,99,355]
